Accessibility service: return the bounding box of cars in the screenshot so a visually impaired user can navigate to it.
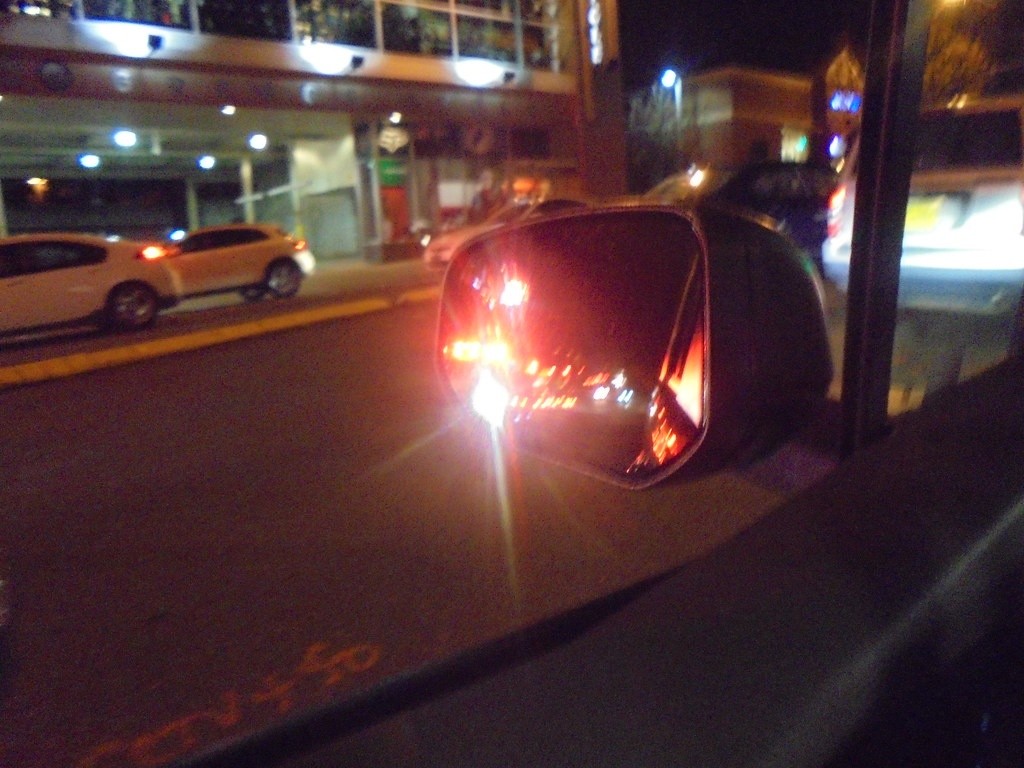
[615,157,835,271]
[0,233,181,352]
[171,223,316,302]
[420,199,609,272]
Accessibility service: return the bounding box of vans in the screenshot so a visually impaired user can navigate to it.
[820,92,1024,326]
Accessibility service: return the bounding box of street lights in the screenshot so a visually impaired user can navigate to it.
[660,69,684,125]
